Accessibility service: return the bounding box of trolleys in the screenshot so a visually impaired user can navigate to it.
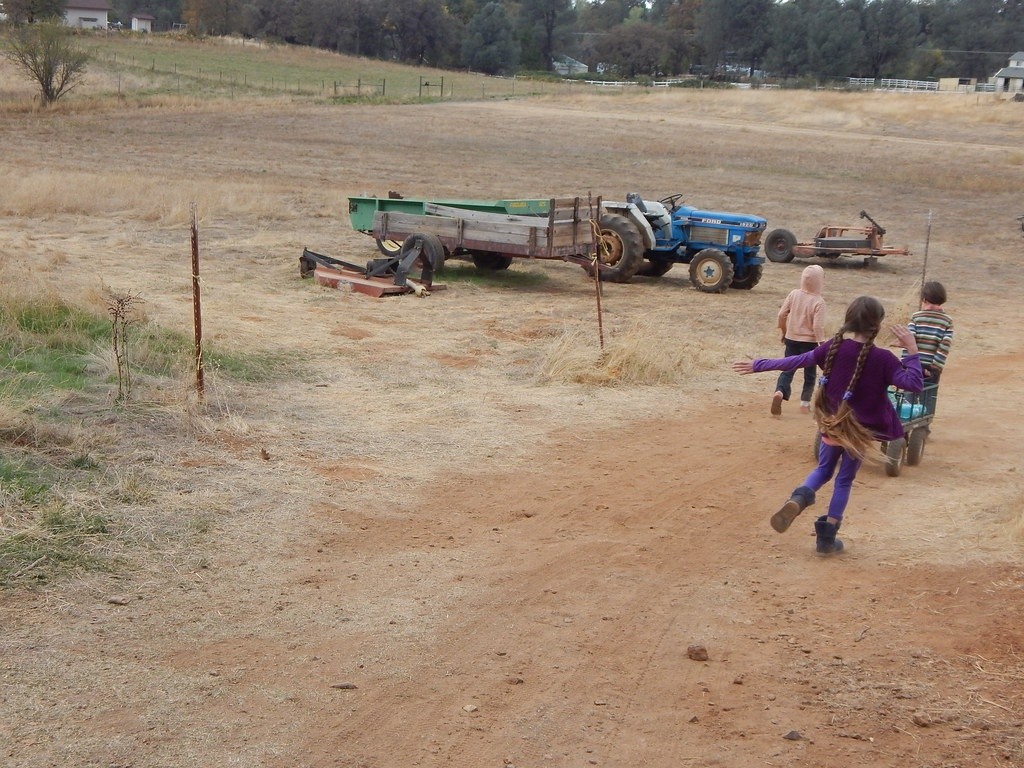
[815,365,939,477]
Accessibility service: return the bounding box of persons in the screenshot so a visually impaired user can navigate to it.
[733,295,924,558]
[771,265,827,415]
[889,281,953,431]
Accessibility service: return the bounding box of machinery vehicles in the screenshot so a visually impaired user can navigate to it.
[347,192,765,296]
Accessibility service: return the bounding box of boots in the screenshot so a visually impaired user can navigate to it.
[770,486,816,533]
[814,514,844,557]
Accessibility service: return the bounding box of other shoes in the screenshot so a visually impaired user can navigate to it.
[800,401,811,413]
[771,391,783,415]
